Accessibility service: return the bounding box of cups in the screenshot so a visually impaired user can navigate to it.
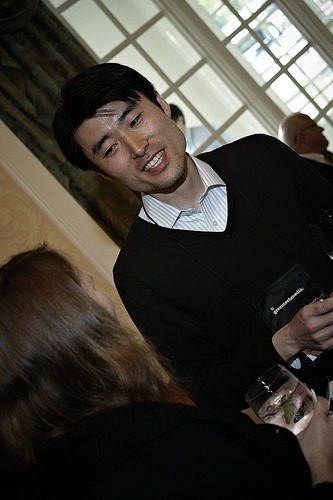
[244,366,319,435]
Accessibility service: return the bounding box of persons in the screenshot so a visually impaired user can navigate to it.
[0,241,333,500]
[52,64,333,414]
[277,112,333,165]
[154,103,233,157]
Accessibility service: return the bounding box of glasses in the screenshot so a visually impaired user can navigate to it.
[294,123,316,146]
